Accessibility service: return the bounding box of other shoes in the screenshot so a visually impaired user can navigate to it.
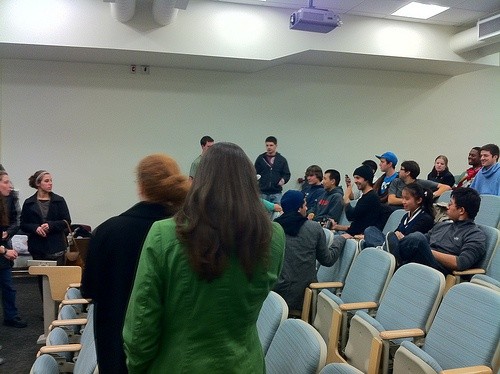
[4,318,28,328]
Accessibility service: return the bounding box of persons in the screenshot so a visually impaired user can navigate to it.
[300,165,324,209]
[453,147,483,188]
[189,136,214,183]
[123,142,286,374]
[307,169,344,231]
[471,144,500,196]
[19,171,71,302]
[274,189,354,312]
[0,165,27,328]
[326,165,380,239]
[261,199,281,212]
[254,136,290,204]
[359,186,487,282]
[428,156,455,188]
[394,182,435,240]
[345,151,451,230]
[81,154,190,374]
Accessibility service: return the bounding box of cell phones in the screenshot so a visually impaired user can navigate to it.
[346,174,350,181]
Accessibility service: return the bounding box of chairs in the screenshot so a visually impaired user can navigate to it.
[256,190,500,374]
[29,282,99,374]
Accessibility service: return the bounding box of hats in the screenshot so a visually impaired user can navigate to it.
[280,190,305,212]
[353,165,374,182]
[375,152,398,165]
[362,160,377,174]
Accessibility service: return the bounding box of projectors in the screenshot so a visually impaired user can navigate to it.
[289,7,342,34]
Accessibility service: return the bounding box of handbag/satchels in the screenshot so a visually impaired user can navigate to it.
[63,251,85,272]
